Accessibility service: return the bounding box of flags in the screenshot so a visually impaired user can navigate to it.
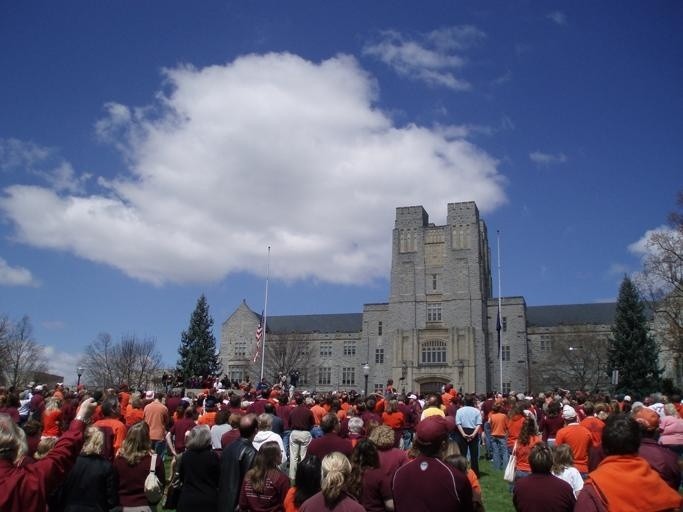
[252,310,263,363]
[495,302,501,359]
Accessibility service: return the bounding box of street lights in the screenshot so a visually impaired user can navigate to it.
[75,366,85,394]
[362,361,370,398]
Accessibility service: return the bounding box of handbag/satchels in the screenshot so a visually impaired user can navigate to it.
[144,472,163,505]
[503,456,518,483]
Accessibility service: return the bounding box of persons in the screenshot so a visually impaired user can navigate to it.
[512,387,683,512]
[440,383,517,478]
[176,375,485,511]
[0,372,198,510]
[280,368,300,387]
